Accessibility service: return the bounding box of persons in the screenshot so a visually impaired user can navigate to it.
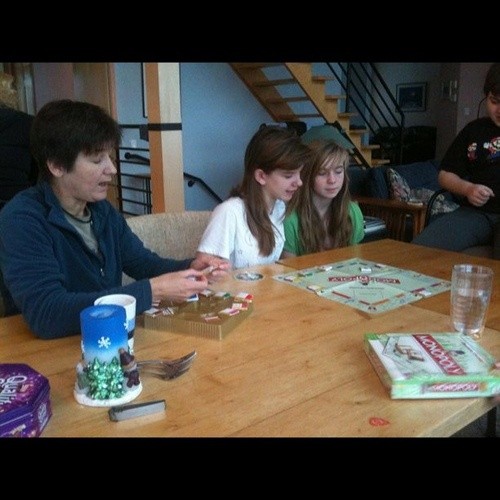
[409,63,500,260]
[196,125,316,276]
[1,98,231,340]
[281,138,365,259]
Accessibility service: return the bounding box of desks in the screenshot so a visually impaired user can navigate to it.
[0,238,500,437]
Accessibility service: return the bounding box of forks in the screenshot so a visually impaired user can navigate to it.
[140,360,194,381]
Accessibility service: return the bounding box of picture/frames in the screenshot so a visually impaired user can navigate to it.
[394,81,428,113]
[140,62,148,118]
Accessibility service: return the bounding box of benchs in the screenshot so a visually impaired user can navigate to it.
[351,190,427,243]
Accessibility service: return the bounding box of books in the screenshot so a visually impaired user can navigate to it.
[362,328,500,400]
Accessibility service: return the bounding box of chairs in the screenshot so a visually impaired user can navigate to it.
[122,210,212,286]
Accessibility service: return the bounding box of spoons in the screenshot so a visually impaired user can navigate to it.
[137,350,196,368]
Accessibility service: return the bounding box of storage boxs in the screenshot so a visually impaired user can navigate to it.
[0,363,53,437]
[362,330,500,401]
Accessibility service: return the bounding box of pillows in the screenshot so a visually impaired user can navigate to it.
[386,167,411,201]
[394,160,439,190]
[354,168,388,199]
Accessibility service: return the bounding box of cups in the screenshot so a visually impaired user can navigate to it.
[94,294,137,354]
[450,264,494,340]
[73,305,143,407]
